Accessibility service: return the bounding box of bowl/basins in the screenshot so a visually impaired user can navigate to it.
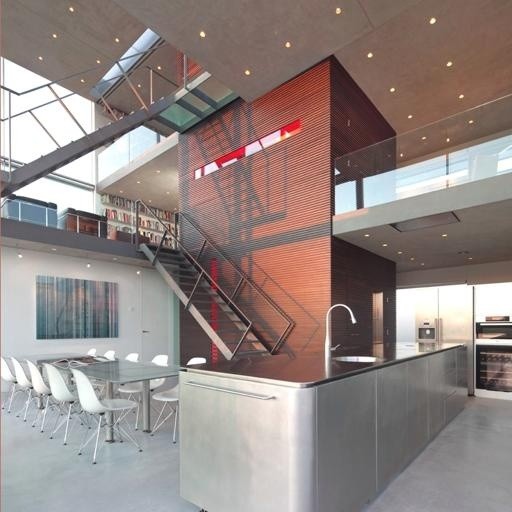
[485,316,509,321]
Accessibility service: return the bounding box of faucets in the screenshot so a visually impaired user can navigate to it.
[323,303,359,357]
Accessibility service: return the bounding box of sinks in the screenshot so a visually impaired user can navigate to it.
[330,354,384,364]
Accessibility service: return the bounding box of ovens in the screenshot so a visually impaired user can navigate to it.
[475,322,512,401]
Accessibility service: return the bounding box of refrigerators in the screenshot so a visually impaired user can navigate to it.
[396,286,473,396]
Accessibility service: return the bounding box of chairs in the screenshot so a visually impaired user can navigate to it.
[0,346,211,469]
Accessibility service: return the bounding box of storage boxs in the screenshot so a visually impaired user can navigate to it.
[57,206,112,238]
[110,230,150,245]
[1,192,59,230]
